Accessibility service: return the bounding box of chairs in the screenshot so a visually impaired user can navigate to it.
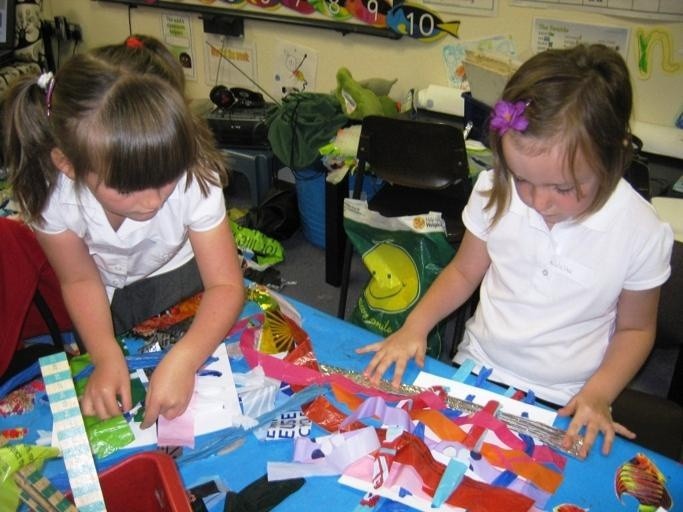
[0,214,73,388]
[338,115,474,360]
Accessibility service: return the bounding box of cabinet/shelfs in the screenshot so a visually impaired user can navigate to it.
[218,145,289,239]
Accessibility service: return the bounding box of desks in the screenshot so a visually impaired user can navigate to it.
[0,276,683,512]
[310,157,683,289]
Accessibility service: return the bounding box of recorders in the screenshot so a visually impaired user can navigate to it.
[190,98,279,151]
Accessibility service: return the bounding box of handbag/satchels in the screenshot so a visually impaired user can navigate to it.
[339,193,454,360]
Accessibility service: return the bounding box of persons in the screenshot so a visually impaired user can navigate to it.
[0,35,247,431]
[356,44,673,460]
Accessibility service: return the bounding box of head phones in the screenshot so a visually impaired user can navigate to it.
[208,82,265,110]
